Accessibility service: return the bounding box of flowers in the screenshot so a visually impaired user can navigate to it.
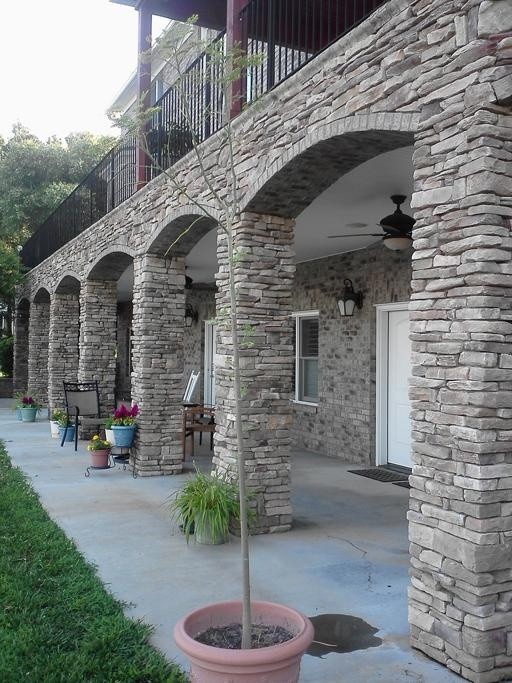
[87,434,115,452]
[110,401,140,425]
[14,387,44,417]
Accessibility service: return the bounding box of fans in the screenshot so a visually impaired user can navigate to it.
[184,265,216,292]
[327,194,415,238]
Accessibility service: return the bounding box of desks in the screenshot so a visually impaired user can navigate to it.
[181,399,202,445]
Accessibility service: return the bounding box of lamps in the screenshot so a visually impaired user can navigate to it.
[384,235,410,251]
[185,302,199,327]
[336,277,364,316]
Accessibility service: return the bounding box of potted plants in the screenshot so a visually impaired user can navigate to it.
[104,14,316,682]
[157,456,264,548]
[102,412,129,454]
[49,405,66,438]
[52,414,75,441]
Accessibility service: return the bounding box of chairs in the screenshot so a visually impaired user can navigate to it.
[182,403,216,462]
[60,379,110,450]
[180,370,201,425]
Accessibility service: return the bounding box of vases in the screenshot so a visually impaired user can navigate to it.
[19,407,37,421]
[111,425,137,446]
[89,449,110,467]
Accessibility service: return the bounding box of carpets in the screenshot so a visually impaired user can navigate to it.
[392,481,411,488]
[347,468,408,482]
